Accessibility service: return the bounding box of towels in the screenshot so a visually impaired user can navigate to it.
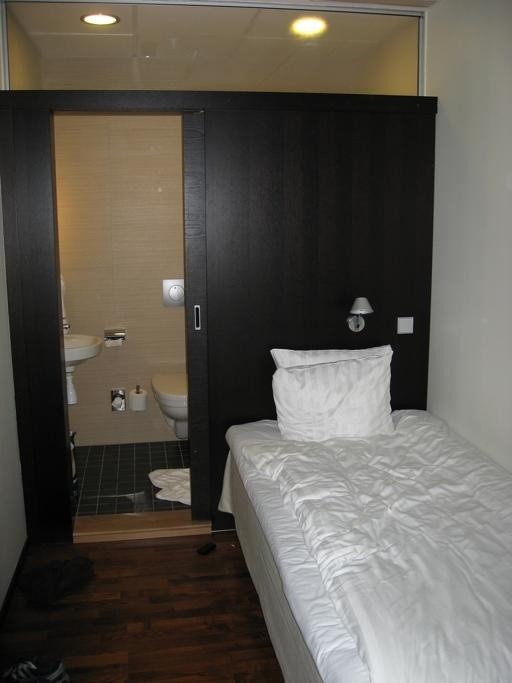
[147,465,191,505]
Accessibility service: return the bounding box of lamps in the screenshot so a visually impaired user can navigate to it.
[347,296,372,332]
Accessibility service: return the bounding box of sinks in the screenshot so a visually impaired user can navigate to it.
[64,335,102,372]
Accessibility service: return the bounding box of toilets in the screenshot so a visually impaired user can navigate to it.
[152,375,189,440]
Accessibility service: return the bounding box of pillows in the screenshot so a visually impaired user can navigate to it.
[273,352,396,441]
[270,344,392,368]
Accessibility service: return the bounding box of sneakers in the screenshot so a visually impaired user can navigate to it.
[0,651,72,683]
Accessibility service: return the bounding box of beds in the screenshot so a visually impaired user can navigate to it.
[216,407,511,681]
